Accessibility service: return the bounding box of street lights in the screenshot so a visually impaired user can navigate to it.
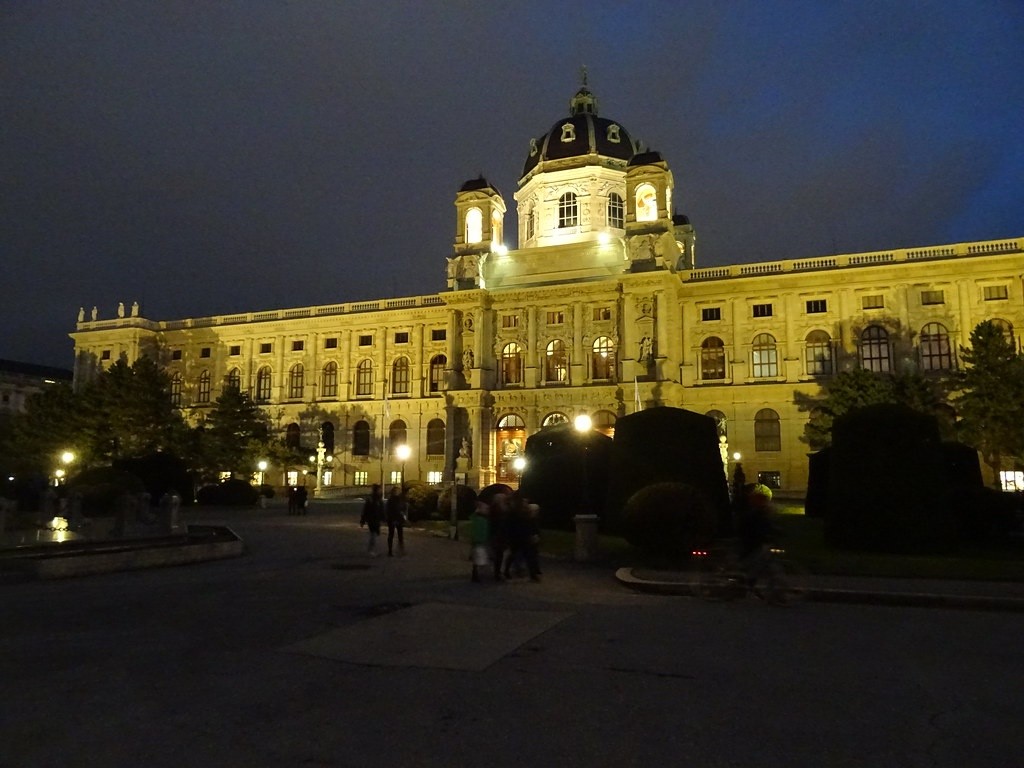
[514,458,525,487]
[260,461,268,484]
[396,445,409,484]
[309,442,332,490]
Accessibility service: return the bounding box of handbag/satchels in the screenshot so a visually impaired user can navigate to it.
[397,512,407,525]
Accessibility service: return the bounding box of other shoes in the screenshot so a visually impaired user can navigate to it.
[531,571,542,580]
[387,552,394,557]
[516,564,526,572]
[494,572,503,583]
[370,552,383,562]
[504,570,511,580]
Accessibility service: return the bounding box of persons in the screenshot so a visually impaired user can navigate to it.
[287,486,308,516]
[385,485,408,558]
[359,483,386,559]
[467,493,542,589]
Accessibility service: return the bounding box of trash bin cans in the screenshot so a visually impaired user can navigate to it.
[572,514,600,563]
